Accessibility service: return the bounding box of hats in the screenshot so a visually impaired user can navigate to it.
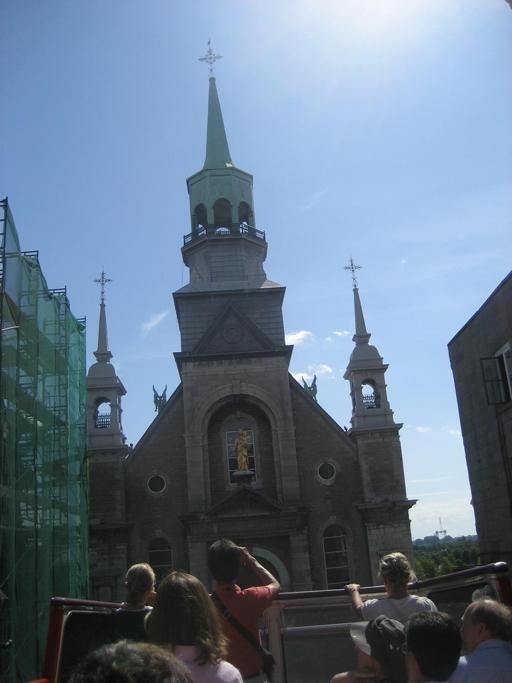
[349,615,408,659]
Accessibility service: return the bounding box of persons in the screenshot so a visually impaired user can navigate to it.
[343,551,439,623]
[93,562,157,647]
[205,537,280,683]
[403,608,463,683]
[233,427,251,472]
[67,636,195,683]
[329,615,406,683]
[445,596,512,683]
[145,566,244,683]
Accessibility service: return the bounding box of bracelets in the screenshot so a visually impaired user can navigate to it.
[351,588,360,592]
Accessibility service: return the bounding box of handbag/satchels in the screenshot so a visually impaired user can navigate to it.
[258,646,276,683]
